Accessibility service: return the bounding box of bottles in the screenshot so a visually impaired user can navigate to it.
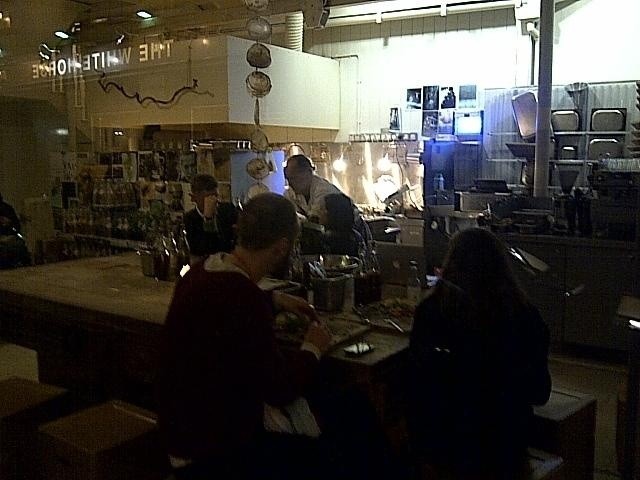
[553,199,575,236]
[406,261,421,306]
[61,178,191,279]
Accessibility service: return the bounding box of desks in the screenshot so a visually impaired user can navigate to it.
[0,244,431,480]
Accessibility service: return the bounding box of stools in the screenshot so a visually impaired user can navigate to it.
[1,375,62,479]
[37,396,161,479]
[534,388,601,479]
[523,444,563,476]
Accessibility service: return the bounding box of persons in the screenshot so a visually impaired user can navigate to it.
[301,193,359,257]
[146,192,332,480]
[183,174,242,256]
[409,227,551,480]
[0,193,27,268]
[282,154,374,256]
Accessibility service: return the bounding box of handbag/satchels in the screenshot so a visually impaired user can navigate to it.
[263,394,321,437]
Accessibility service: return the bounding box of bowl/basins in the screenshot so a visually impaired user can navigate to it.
[317,254,363,274]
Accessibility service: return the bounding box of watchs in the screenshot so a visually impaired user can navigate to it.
[203,214,215,221]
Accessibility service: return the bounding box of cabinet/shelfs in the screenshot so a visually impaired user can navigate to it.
[484,105,633,195]
[503,239,631,354]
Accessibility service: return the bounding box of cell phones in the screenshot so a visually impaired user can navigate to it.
[345,341,375,354]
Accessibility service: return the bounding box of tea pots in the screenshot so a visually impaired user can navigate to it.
[307,274,352,311]
